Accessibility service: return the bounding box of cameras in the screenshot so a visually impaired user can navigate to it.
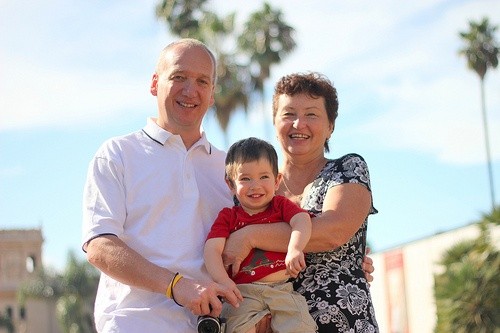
[196,295,227,333]
